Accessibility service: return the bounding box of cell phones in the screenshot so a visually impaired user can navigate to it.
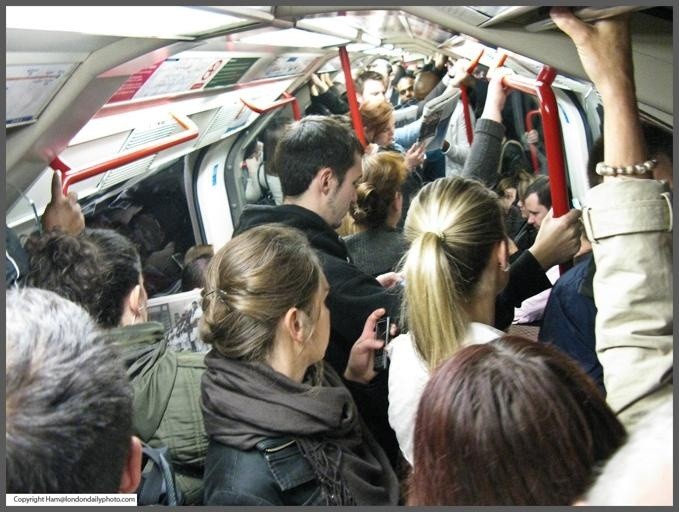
[374,316,391,372]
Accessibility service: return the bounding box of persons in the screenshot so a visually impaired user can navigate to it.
[3,51,674,505]
[396,6,673,504]
